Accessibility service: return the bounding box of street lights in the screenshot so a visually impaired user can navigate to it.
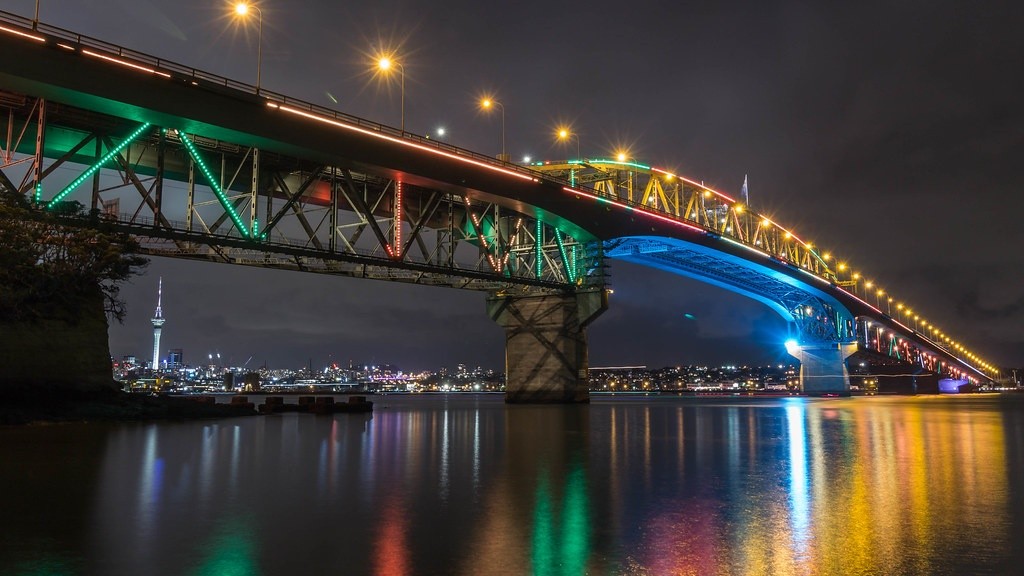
[939,333,999,380]
[905,311,911,327]
[921,320,926,334]
[914,315,919,331]
[481,98,506,164]
[840,264,854,294]
[823,254,837,278]
[854,274,867,302]
[928,325,932,339]
[934,330,938,343]
[379,56,406,137]
[806,243,820,274]
[666,172,685,221]
[618,154,638,208]
[878,289,890,316]
[898,305,903,322]
[559,130,581,190]
[888,298,893,317]
[233,1,263,94]
[866,282,880,309]
